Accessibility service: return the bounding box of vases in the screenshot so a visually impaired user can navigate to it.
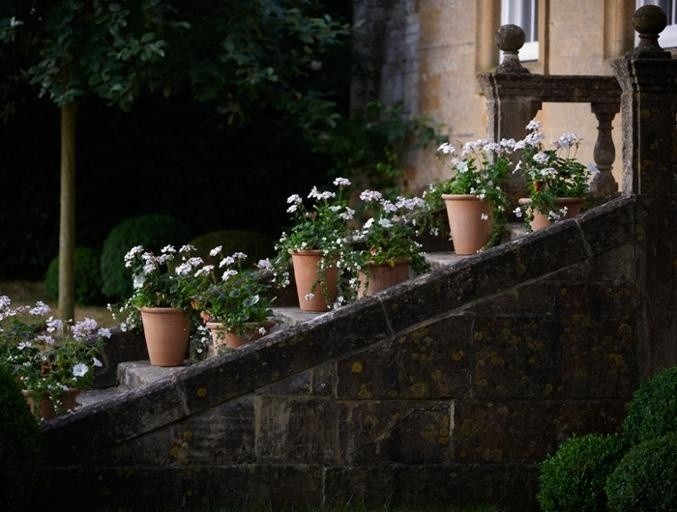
[136,306,192,367]
[518,198,585,231]
[357,259,409,298]
[442,194,495,255]
[288,249,341,310]
[207,323,274,355]
[20,389,78,418]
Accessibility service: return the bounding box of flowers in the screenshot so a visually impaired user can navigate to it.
[175,245,290,342]
[513,120,599,222]
[423,140,521,257]
[274,177,354,265]
[304,188,430,308]
[0,295,111,426]
[107,245,206,334]
